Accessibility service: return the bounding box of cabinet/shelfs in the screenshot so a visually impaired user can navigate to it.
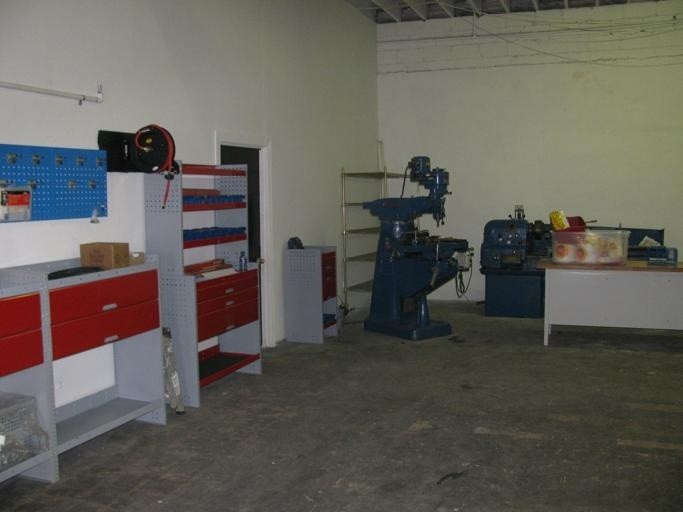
[1,255,168,484]
[340,167,421,323]
[284,245,339,344]
[144,160,263,408]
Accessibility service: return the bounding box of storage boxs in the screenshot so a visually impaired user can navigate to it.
[80,242,130,269]
[550,229,632,266]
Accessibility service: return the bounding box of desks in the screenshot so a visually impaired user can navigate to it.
[537,261,683,346]
[479,266,544,319]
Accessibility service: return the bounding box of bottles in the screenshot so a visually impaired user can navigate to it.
[0,180,9,222]
[239,251,248,272]
